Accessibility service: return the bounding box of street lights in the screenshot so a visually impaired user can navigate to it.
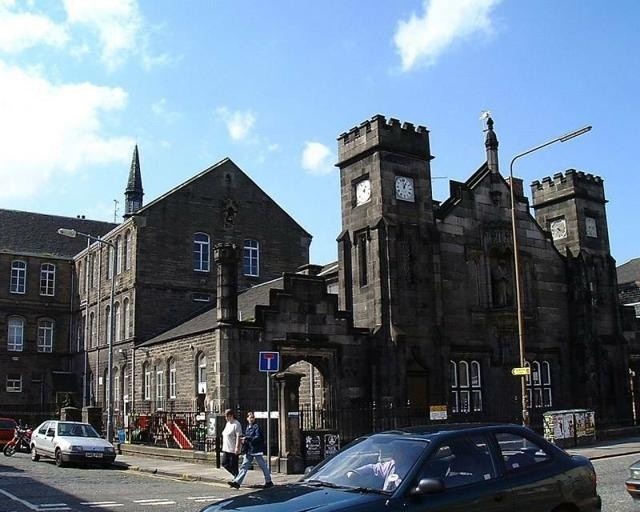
[508,122,595,428]
[54,228,118,447]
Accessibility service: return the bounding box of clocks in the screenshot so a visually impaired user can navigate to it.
[395,175,415,204]
[356,181,371,201]
[551,219,566,238]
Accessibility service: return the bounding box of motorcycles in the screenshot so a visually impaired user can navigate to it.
[2,418,33,457]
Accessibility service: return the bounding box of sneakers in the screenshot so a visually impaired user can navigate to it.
[228,480,240,489]
[263,481,273,489]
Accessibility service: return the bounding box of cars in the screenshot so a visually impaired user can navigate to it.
[198,418,603,512]
[29,418,117,469]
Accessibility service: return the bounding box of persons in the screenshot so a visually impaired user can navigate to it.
[221,409,274,490]
